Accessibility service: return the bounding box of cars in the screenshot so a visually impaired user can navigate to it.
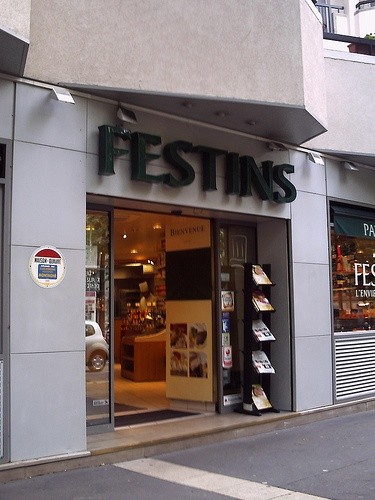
[85,321,109,373]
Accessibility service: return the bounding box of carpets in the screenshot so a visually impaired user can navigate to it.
[87,408,202,428]
[86,397,147,417]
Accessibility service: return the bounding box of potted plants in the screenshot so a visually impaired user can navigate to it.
[348,32,375,57]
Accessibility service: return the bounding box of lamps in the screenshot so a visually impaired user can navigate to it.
[308,153,324,166]
[269,142,288,151]
[344,161,359,172]
[116,107,139,125]
[51,88,75,104]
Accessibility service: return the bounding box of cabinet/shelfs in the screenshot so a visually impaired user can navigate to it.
[232,261,280,417]
[121,337,166,380]
